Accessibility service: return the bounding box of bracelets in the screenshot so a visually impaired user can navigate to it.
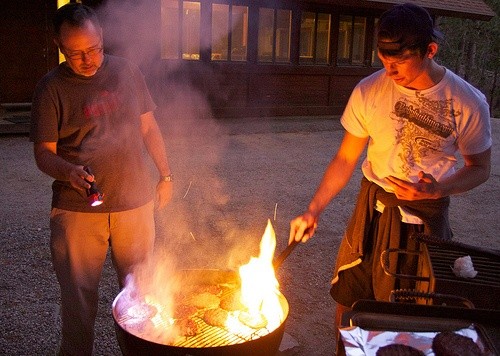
[160,174,174,181]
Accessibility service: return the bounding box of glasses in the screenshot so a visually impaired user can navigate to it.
[60,30,102,60]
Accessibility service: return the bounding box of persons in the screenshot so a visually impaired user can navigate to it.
[28,3,174,356]
[288,3,492,356]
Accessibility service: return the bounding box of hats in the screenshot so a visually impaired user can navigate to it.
[375,3,444,58]
